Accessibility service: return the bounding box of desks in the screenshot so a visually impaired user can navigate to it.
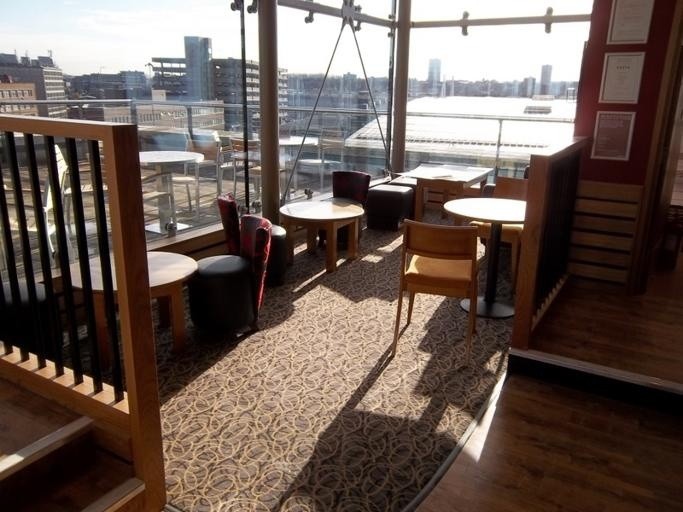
[394,161,494,226]
[138,150,205,235]
[443,197,527,318]
[71,251,199,367]
[278,198,365,274]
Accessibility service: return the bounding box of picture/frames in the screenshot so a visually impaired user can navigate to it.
[590,110,637,161]
[597,51,646,105]
[605,0,655,45]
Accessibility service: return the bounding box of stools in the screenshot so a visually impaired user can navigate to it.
[366,183,415,231]
[387,175,425,217]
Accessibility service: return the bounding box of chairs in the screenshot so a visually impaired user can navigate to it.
[0,277,63,360]
[85,139,176,248]
[467,174,528,299]
[318,170,371,247]
[480,164,530,197]
[391,218,482,367]
[183,125,345,217]
[217,193,294,287]
[44,144,108,265]
[186,213,273,338]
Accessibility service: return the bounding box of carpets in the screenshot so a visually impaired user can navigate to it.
[49,220,517,512]
